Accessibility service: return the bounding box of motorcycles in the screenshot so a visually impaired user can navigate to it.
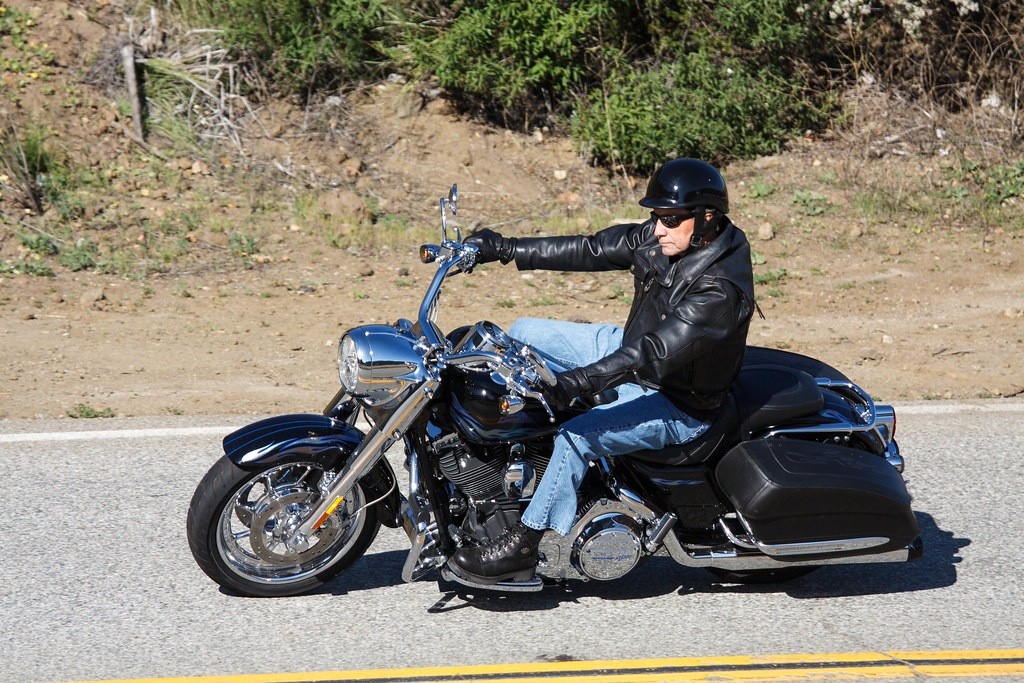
[184,183,927,599]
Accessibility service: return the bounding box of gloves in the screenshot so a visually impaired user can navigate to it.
[462,228,518,265]
[539,367,592,406]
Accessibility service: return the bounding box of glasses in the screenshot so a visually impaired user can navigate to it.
[650,211,695,229]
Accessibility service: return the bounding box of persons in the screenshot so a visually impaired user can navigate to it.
[448,157,754,587]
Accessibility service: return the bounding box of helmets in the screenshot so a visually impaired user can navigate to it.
[639,158,729,213]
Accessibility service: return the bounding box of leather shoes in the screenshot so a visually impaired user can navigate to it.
[447,519,545,584]
